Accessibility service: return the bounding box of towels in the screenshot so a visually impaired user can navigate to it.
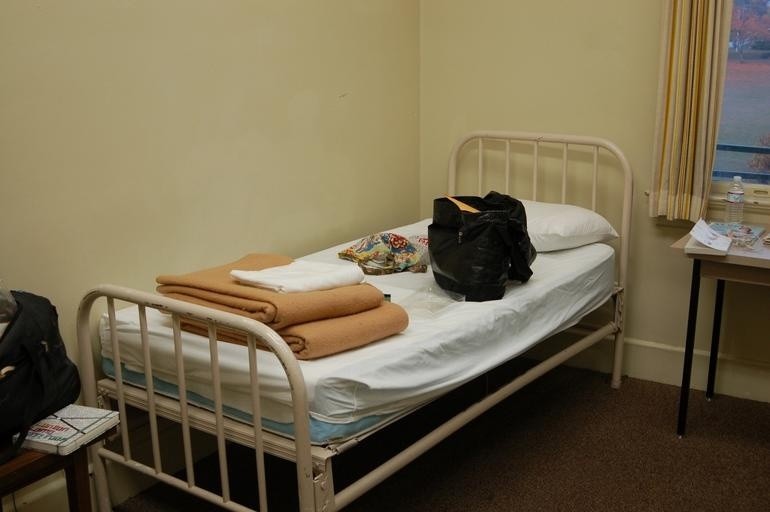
[229,260,364,294]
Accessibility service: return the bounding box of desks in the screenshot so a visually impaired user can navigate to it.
[1,446,92,512]
[669,219,770,437]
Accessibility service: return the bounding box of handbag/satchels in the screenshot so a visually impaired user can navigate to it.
[0,290,80,466]
[428,191,536,301]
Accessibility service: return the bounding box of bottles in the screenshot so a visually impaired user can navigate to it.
[725,176,745,223]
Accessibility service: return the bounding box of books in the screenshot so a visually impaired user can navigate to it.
[684,217,732,256]
[12,403,120,457]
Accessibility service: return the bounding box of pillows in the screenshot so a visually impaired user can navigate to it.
[516,198,620,253]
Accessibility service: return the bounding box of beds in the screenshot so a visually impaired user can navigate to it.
[75,128,634,512]
